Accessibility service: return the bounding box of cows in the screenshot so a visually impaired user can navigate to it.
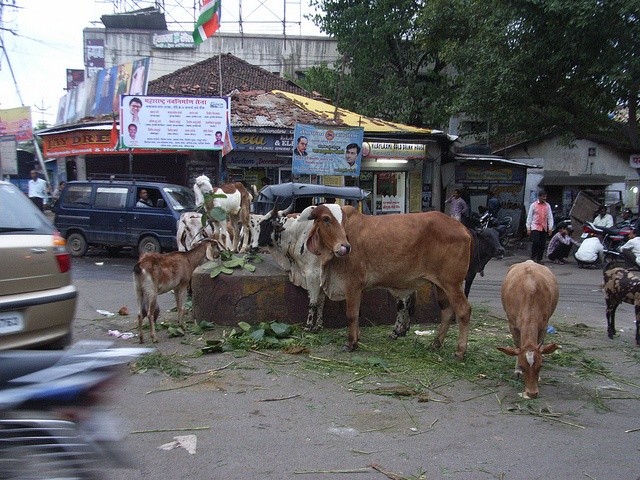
[249,195,411,338]
[464,227,506,299]
[603,266,640,345]
[297,204,474,362]
[497,259,559,401]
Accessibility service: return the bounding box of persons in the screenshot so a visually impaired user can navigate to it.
[546,225,581,265]
[87,75,97,116]
[294,136,308,156]
[116,64,129,95]
[616,229,640,271]
[526,191,554,265]
[486,192,499,218]
[615,208,640,229]
[478,206,483,213]
[136,189,154,207]
[444,189,468,222]
[128,98,143,122]
[76,82,87,118]
[58,95,66,122]
[214,131,224,146]
[52,181,66,202]
[574,233,607,269]
[282,176,290,182]
[251,177,269,201]
[98,69,112,113]
[592,205,614,229]
[127,124,139,145]
[67,90,75,119]
[130,60,145,95]
[342,143,360,169]
[325,198,336,204]
[28,169,48,212]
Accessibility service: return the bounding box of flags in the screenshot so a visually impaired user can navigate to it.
[192,0,222,44]
[221,121,236,158]
[109,117,119,151]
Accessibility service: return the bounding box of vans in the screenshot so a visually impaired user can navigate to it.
[55,181,199,257]
[0,180,78,352]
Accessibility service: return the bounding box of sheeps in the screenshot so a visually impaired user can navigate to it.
[176,212,263,251]
[193,175,249,253]
[131,238,222,344]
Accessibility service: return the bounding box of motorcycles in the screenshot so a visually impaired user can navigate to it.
[581,218,640,268]
[2,348,154,480]
[475,210,514,248]
